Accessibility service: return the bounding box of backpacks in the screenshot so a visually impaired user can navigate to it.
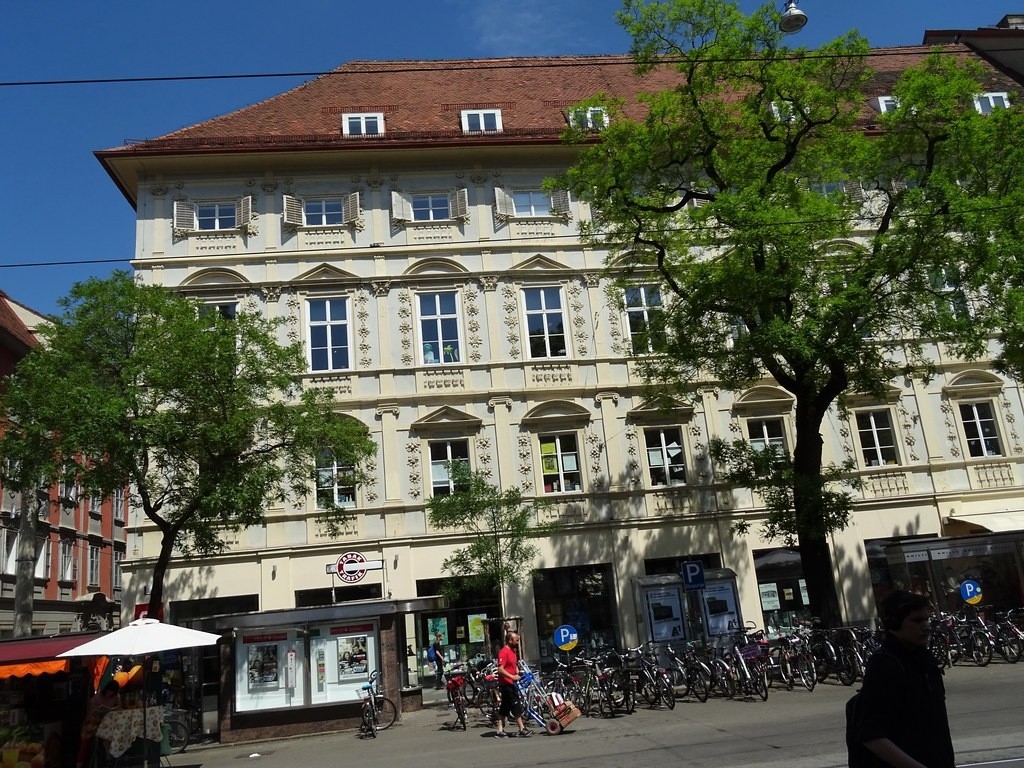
[427,645,438,662]
[845,648,907,768]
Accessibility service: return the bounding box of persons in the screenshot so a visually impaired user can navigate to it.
[341,645,366,668]
[846,589,955,768]
[494,631,532,738]
[76,680,122,768]
[433,633,446,690]
[250,651,277,681]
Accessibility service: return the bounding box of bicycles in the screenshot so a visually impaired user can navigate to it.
[355,669,397,739]
[435,600,1024,731]
[163,718,190,755]
[188,698,202,734]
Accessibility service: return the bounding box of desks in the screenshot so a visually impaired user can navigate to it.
[95,705,165,768]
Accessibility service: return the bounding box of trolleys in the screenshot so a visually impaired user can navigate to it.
[512,669,566,735]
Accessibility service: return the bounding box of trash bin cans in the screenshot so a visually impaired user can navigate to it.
[400,684,424,713]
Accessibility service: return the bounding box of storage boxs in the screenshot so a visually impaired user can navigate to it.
[544,692,580,727]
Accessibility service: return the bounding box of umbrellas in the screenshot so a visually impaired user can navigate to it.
[55,618,222,768]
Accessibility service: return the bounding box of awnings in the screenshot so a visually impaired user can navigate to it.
[0,630,100,678]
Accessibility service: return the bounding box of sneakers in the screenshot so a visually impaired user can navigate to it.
[434,686,444,690]
[494,732,509,739]
[519,730,533,737]
